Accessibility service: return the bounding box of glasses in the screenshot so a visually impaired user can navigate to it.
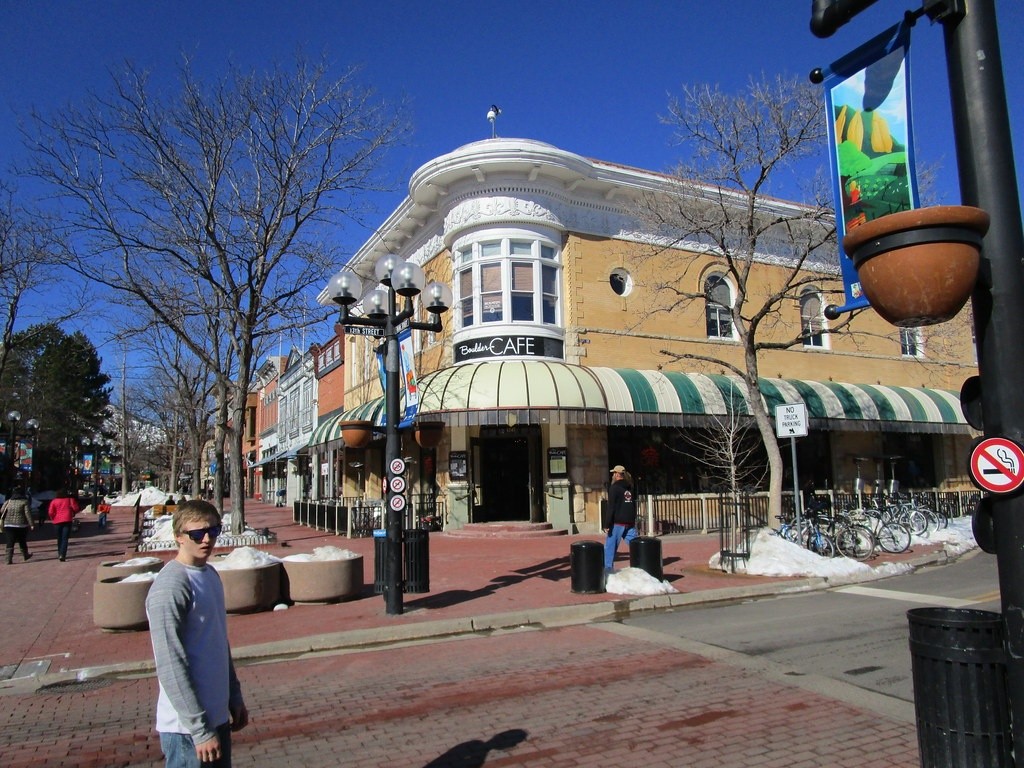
[182,523,221,544]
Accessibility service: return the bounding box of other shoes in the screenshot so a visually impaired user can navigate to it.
[58,552,66,562]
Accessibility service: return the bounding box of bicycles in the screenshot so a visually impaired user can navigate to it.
[775,491,949,562]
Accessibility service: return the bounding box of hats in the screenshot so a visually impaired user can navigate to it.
[610,465,627,474]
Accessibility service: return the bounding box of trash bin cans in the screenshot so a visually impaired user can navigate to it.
[276,490,286,504]
[629,536,663,583]
[907,607,1009,767]
[403,529,430,593]
[374,536,387,593]
[570,540,604,594]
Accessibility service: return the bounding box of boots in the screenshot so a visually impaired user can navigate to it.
[20,548,33,560]
[6,548,12,564]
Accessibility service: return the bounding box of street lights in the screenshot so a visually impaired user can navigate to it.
[327,253,453,617]
[82,432,114,514]
[0,410,40,502]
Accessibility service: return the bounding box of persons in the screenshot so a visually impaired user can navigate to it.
[604,465,640,575]
[47,490,80,562]
[0,485,35,565]
[177,495,188,505]
[144,499,250,768]
[805,479,816,500]
[96,499,111,530]
[165,495,175,505]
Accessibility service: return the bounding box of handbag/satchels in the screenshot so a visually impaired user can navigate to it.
[0,518,4,533]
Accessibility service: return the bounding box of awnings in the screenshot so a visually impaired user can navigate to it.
[247,443,311,468]
[307,360,807,458]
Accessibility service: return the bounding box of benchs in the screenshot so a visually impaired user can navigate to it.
[153,504,179,515]
